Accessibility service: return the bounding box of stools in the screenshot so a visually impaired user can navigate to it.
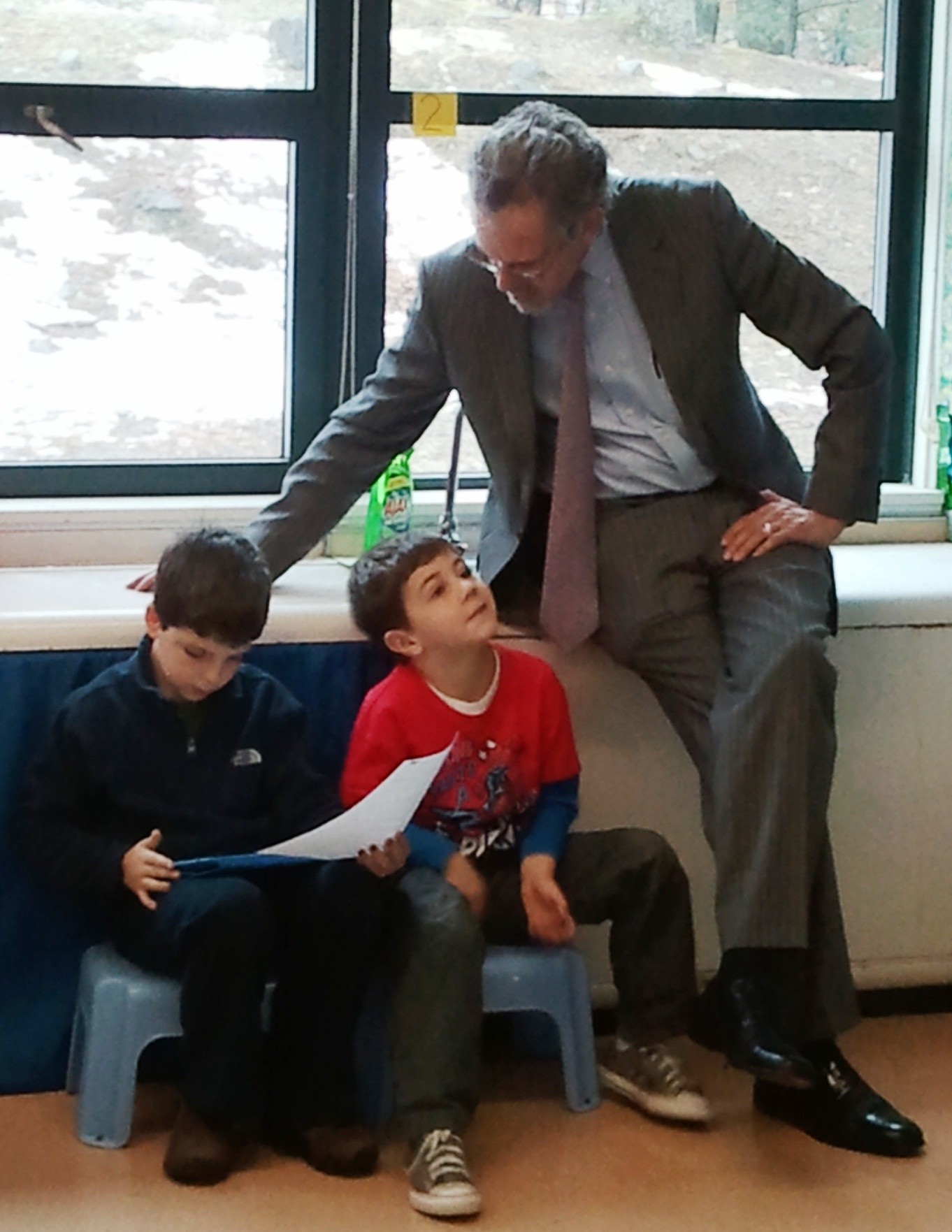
[347,934,597,1125]
[62,943,277,1150]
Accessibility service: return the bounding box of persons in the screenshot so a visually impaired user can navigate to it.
[336,535,712,1216]
[28,526,382,1184]
[127,103,925,1156]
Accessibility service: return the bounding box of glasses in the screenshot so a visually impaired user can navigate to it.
[463,226,576,278]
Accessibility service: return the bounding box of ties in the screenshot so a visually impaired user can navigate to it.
[539,273,601,654]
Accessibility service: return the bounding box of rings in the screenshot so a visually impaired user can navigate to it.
[764,523,774,536]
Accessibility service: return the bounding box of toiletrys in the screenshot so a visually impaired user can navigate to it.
[361,446,416,553]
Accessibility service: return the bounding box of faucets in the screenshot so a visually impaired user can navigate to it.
[436,403,469,557]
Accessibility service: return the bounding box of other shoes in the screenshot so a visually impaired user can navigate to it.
[258,1122,378,1177]
[165,1107,229,1185]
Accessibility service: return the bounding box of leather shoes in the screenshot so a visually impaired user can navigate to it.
[752,1038,925,1156]
[689,975,814,1088]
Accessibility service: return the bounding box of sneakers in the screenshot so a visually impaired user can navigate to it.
[407,1129,480,1217]
[595,1042,712,1123]
